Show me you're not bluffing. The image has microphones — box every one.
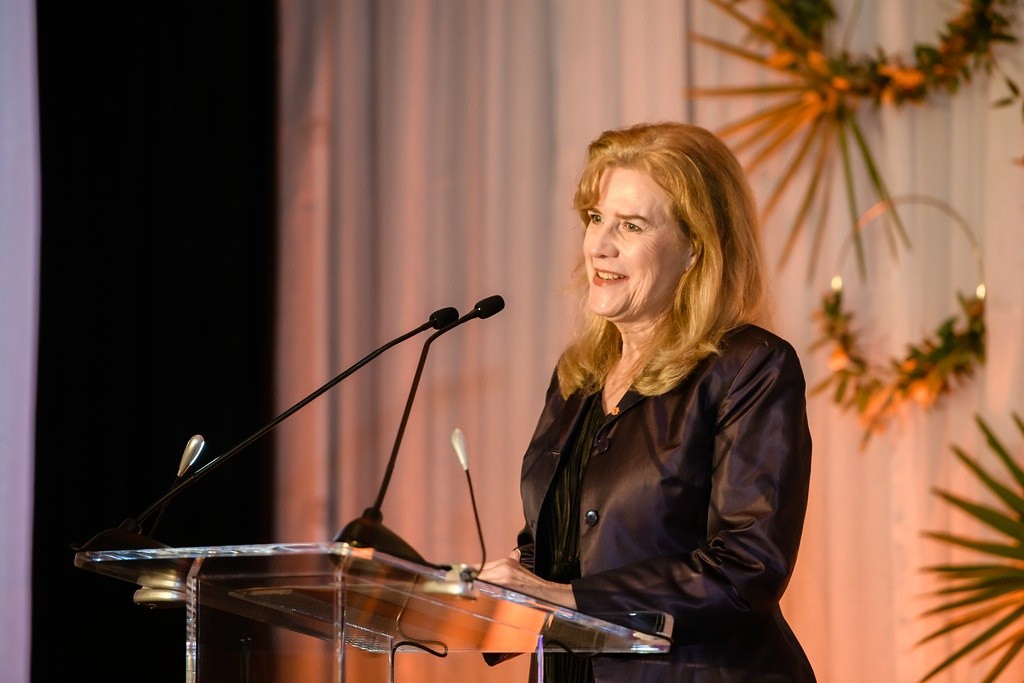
[78,305,460,567]
[329,294,505,580]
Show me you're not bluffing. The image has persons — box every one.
[458,120,822,683]
